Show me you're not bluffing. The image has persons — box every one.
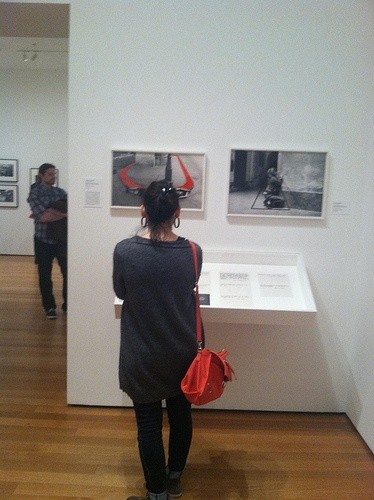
[27,162,68,320]
[112,180,206,500]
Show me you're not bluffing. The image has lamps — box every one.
[23,52,38,62]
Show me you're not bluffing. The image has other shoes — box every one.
[46,309,57,319]
[145,467,182,497]
[127,489,169,500]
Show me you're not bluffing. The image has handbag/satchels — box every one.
[179,347,238,406]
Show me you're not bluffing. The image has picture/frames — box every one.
[109,148,207,212]
[225,147,330,219]
[29,168,59,188]
[0,185,18,208]
[0,159,18,182]
